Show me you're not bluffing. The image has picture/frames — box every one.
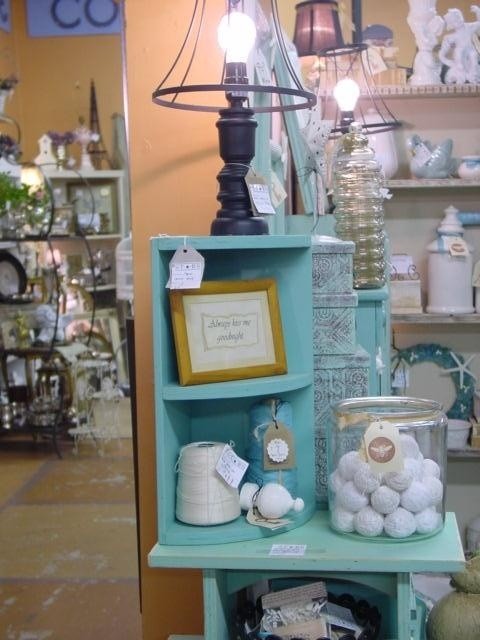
[165,278,286,386]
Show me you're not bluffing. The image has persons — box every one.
[436,4,480,84]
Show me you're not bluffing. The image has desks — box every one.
[148,510,468,639]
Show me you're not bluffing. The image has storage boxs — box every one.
[313,235,373,510]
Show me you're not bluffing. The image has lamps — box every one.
[151,0,318,235]
[318,43,401,136]
[293,1,345,57]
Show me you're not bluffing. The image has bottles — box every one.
[331,121,387,290]
[327,395,448,544]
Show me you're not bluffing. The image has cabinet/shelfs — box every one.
[359,290,393,398]
[284,79,478,461]
